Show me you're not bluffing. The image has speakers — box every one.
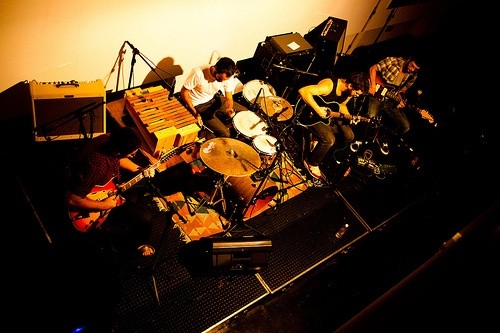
[318,17,348,68]
[200,237,273,276]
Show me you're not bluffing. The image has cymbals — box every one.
[198,136,261,178]
[260,97,294,120]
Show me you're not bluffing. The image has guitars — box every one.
[296,91,369,127]
[357,63,434,122]
[69,140,193,234]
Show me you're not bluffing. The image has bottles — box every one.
[335,223,349,238]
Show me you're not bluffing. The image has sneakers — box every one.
[303,159,322,180]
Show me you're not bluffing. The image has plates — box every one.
[138,245,157,256]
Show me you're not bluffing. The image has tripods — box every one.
[223,125,285,237]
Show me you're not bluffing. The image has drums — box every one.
[242,77,276,104]
[254,134,281,168]
[230,109,272,137]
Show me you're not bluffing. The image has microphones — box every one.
[168,79,177,100]
[254,89,262,104]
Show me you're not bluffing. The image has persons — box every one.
[181,57,249,139]
[351,55,422,156]
[298,71,369,180]
[65,128,156,257]
